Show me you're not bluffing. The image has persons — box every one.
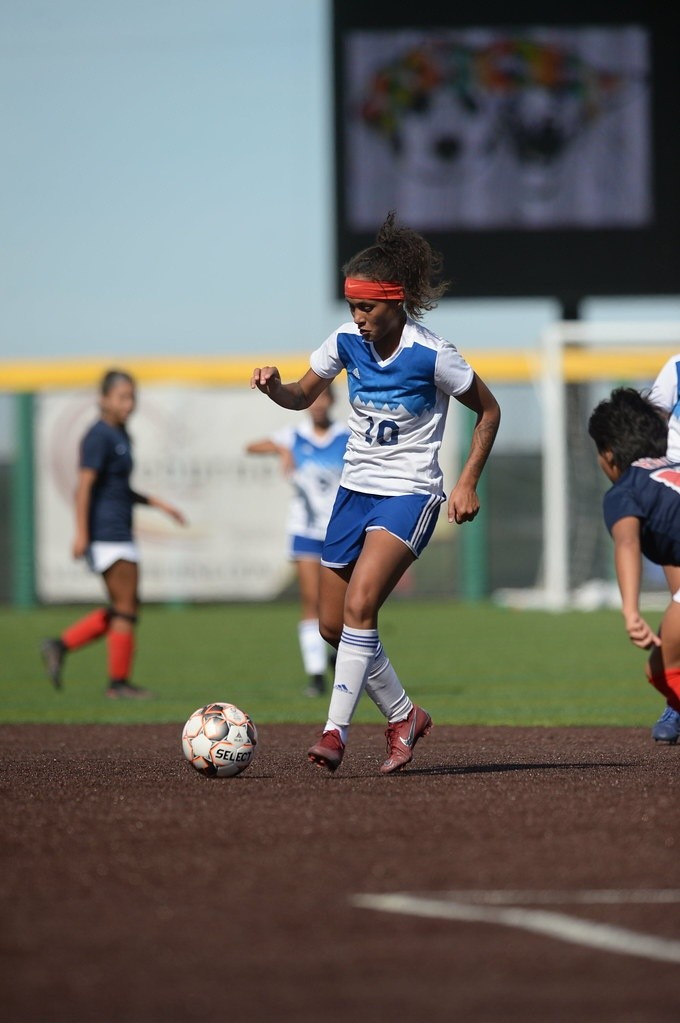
[250,208,502,777]
[643,354,680,744]
[245,385,353,697]
[39,371,187,699]
[588,386,680,719]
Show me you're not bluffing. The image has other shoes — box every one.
[109,683,153,700]
[41,642,66,688]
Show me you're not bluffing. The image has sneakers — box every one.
[308,730,345,774]
[651,705,680,746]
[381,705,434,774]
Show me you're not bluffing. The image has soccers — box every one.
[181,701,260,778]
